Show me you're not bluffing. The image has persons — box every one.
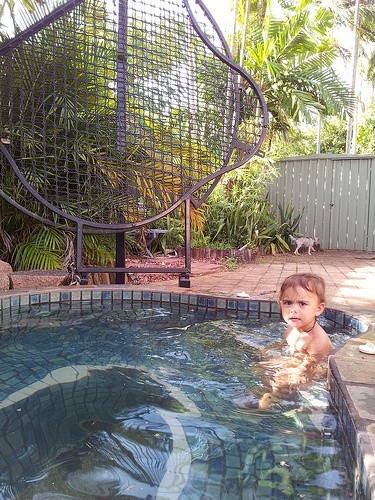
[236,273,335,409]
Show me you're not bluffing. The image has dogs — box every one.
[288,235,320,256]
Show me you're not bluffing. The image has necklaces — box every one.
[300,321,316,333]
